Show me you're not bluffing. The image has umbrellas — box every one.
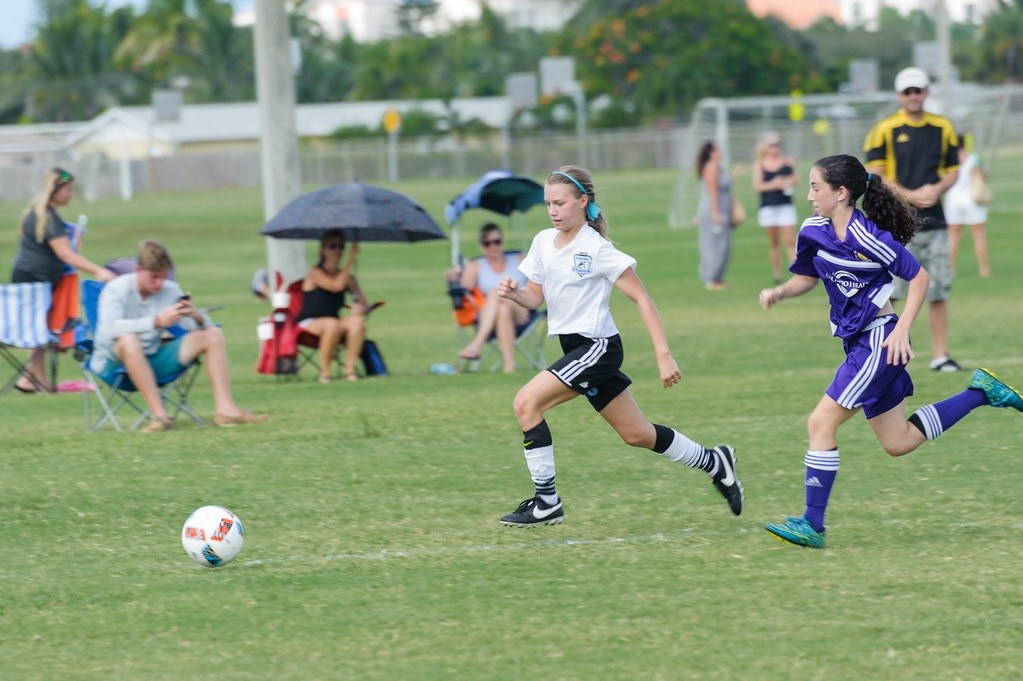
[259,178,446,243]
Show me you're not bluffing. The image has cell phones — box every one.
[179,296,189,300]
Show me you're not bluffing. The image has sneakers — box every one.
[499,495,564,529]
[967,368,1023,412]
[711,445,746,516]
[764,515,826,549]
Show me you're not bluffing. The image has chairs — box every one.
[448,250,548,373]
[0,256,222,433]
[269,279,386,382]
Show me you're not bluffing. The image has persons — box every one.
[752,132,798,284]
[695,139,745,289]
[446,223,532,373]
[864,66,961,374]
[90,240,268,434]
[760,154,1023,549]
[497,164,745,527]
[941,137,992,278]
[252,268,268,303]
[11,167,116,392]
[296,230,369,382]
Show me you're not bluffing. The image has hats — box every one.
[894,67,928,92]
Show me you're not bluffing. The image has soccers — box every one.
[181,505,244,567]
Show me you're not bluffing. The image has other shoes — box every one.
[930,360,961,371]
[457,351,479,359]
[140,417,174,434]
[214,409,270,428]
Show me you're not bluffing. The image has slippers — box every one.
[14,384,36,393]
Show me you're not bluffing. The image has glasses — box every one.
[323,243,346,250]
[482,239,501,247]
